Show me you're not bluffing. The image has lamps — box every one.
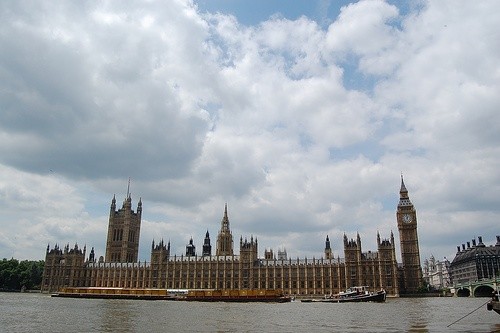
[402,213,413,223]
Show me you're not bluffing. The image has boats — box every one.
[51,292,60,297]
[186,289,292,301]
[301,299,312,302]
[322,286,387,303]
[61,287,170,298]
